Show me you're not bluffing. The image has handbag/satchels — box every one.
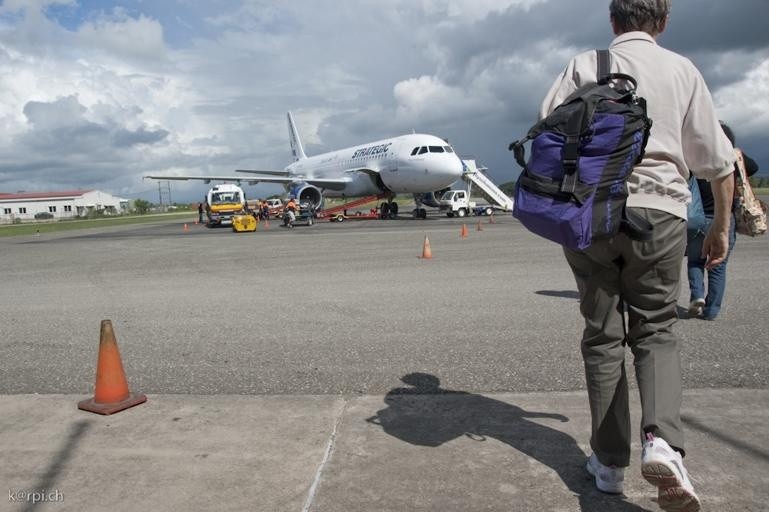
[687,175,707,239]
[732,147,769,237]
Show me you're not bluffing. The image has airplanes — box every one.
[138,108,488,221]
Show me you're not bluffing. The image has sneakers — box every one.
[640,433,702,511]
[586,450,626,494]
[689,298,704,317]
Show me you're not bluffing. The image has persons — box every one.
[198,203,205,223]
[259,198,297,229]
[686,121,758,321]
[539,0,737,511]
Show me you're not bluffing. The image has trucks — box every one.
[203,183,249,228]
[264,198,283,218]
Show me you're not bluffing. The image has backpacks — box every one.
[508,72,653,250]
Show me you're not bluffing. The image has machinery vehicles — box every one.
[438,157,514,218]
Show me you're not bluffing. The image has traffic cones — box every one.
[419,234,435,260]
[458,222,471,239]
[73,315,150,419]
[487,214,495,224]
[476,218,482,231]
[182,223,189,231]
[194,219,198,224]
